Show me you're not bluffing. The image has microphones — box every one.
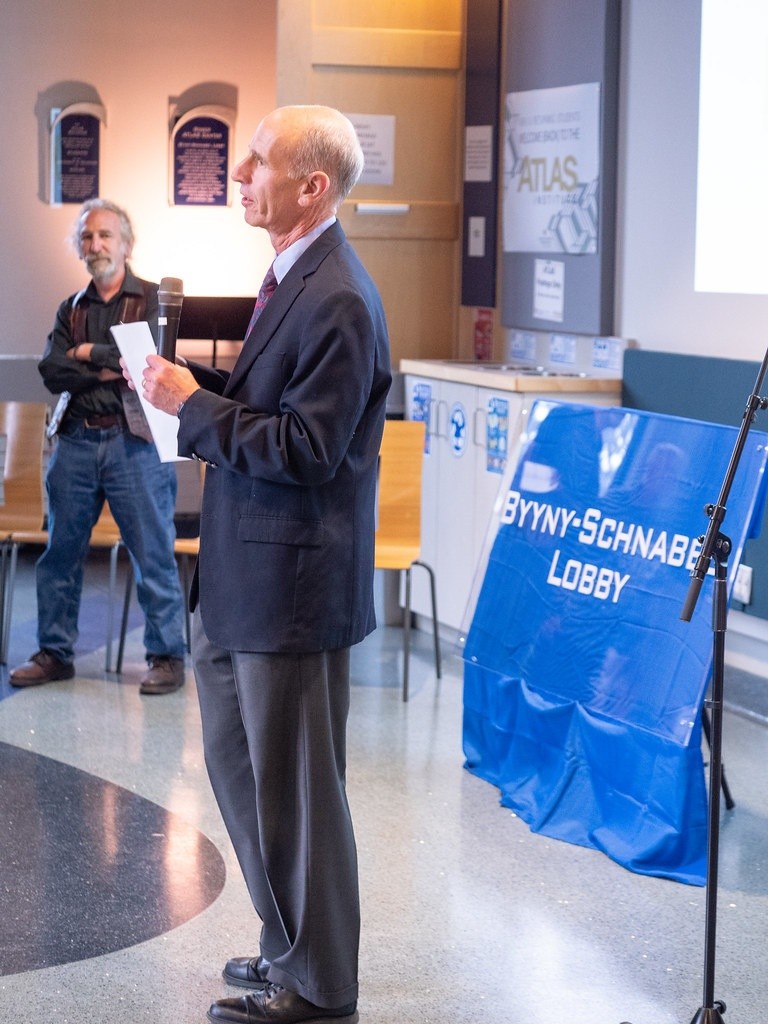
[157,277,185,367]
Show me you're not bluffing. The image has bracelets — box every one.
[174,399,187,420]
[72,341,86,360]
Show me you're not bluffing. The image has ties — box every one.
[243,263,278,343]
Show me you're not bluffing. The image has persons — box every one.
[118,106,391,1024]
[7,197,187,696]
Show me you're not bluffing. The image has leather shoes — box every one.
[8,650,74,686]
[206,981,359,1023]
[139,656,185,694]
[222,954,271,988]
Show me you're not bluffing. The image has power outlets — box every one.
[732,564,752,604]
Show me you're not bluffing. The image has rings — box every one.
[141,380,148,387]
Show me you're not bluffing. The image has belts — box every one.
[83,413,127,429]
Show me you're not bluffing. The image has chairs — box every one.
[0,401,440,703]
